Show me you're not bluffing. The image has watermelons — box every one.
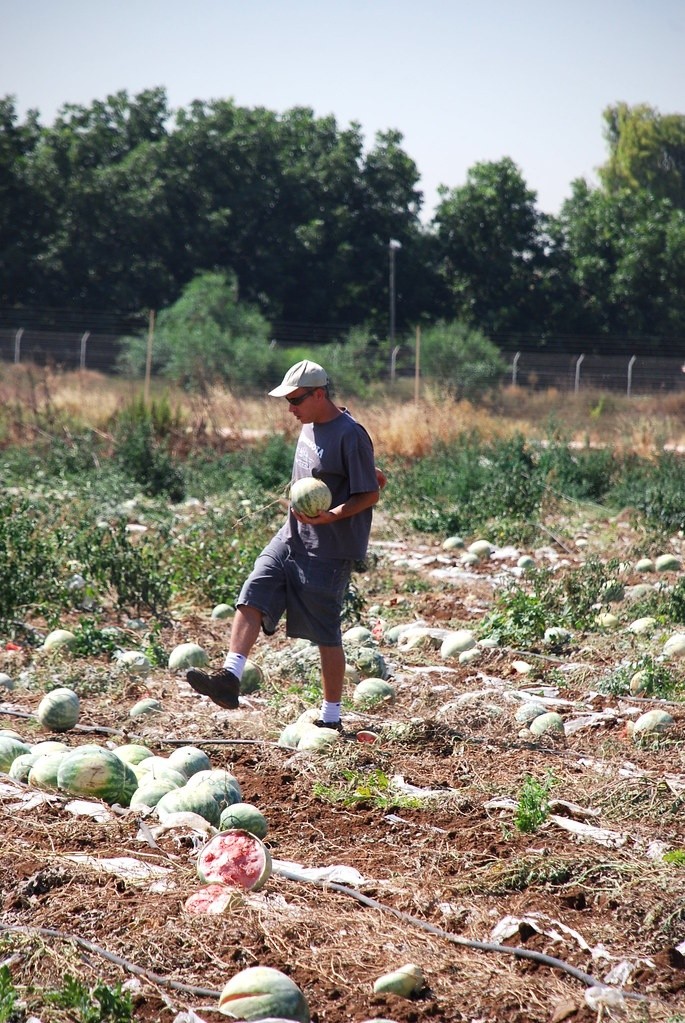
[0,477,685,1023]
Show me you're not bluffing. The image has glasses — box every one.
[285,387,318,406]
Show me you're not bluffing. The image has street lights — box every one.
[387,238,403,353]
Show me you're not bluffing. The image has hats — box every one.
[267,359,328,398]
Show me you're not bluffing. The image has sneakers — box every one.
[186,668,239,709]
[295,717,344,750]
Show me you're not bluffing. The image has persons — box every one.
[186,359,383,732]
